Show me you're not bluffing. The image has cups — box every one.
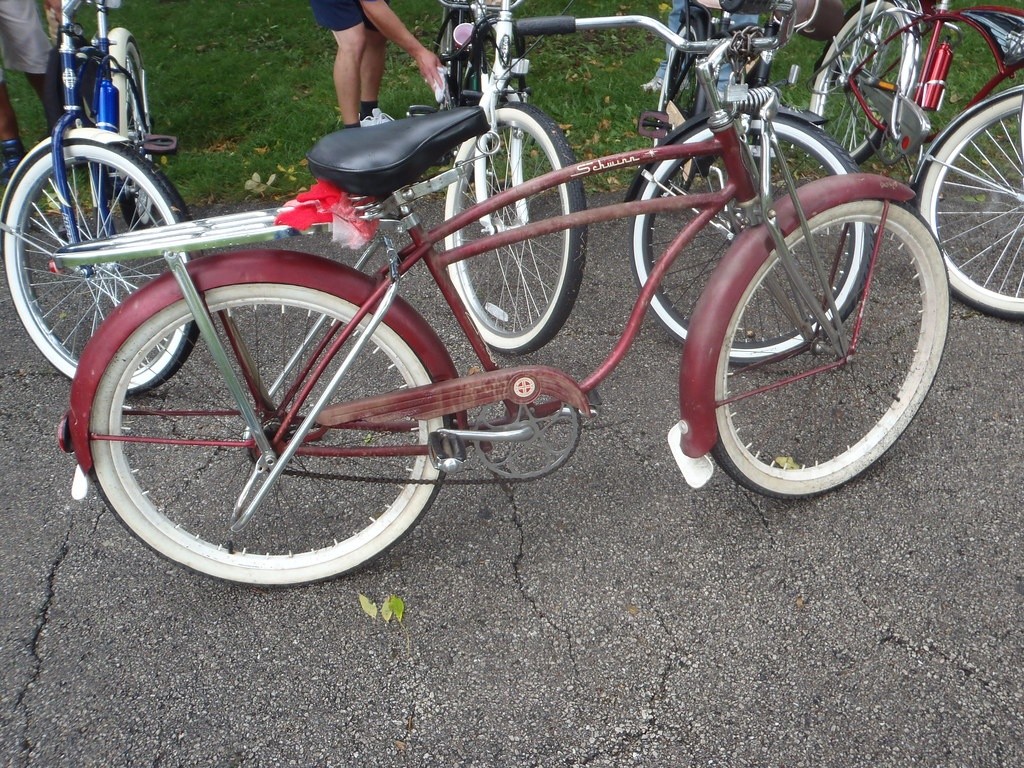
[453,23,474,48]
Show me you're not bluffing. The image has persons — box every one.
[0,0,61,186]
[309,0,444,129]
[641,0,759,102]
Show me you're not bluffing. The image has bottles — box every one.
[918,34,953,111]
[94,77,120,133]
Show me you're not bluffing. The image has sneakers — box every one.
[641,77,663,90]
[2,139,24,183]
[359,108,395,127]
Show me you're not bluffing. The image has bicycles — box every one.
[806,1,1024,322]
[404,1,588,356]
[621,0,873,368]
[46,15,951,592]
[0,0,207,398]
